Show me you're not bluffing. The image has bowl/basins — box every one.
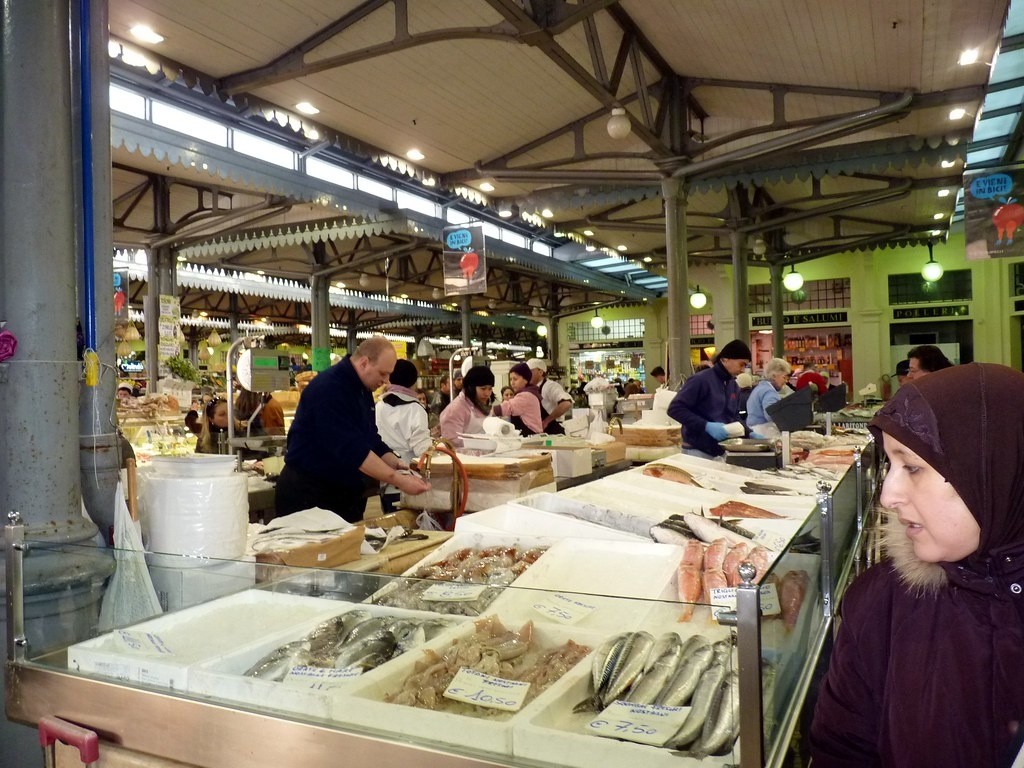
[719,438,775,450]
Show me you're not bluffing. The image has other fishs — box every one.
[641,430,871,633]
[570,630,777,760]
[243,609,458,685]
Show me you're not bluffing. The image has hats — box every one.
[792,368,802,375]
[389,359,418,388]
[453,369,462,380]
[736,373,752,388]
[890,359,909,377]
[820,371,828,377]
[720,340,752,362]
[462,366,494,387]
[119,382,133,394]
[527,358,547,373]
[509,363,532,381]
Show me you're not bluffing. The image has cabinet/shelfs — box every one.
[754,336,834,377]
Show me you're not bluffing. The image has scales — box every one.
[723,386,814,471]
[226,337,291,451]
[448,348,491,402]
[814,383,846,436]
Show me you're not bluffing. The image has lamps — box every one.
[784,263,804,291]
[607,107,632,139]
[690,284,706,308]
[921,47,992,281]
[105,23,603,338]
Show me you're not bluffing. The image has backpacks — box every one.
[234,394,276,461]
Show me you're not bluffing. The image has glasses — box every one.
[207,396,222,412]
[905,368,921,373]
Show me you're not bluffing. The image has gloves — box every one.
[748,432,763,439]
[705,421,728,441]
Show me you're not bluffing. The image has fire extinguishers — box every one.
[882,374,891,401]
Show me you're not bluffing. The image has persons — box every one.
[274,336,431,525]
[115,356,846,514]
[668,340,769,456]
[802,362,1024,768]
[891,346,954,387]
[746,358,791,437]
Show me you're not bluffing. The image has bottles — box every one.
[783,333,851,352]
[784,354,840,377]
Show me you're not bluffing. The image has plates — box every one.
[150,455,238,476]
